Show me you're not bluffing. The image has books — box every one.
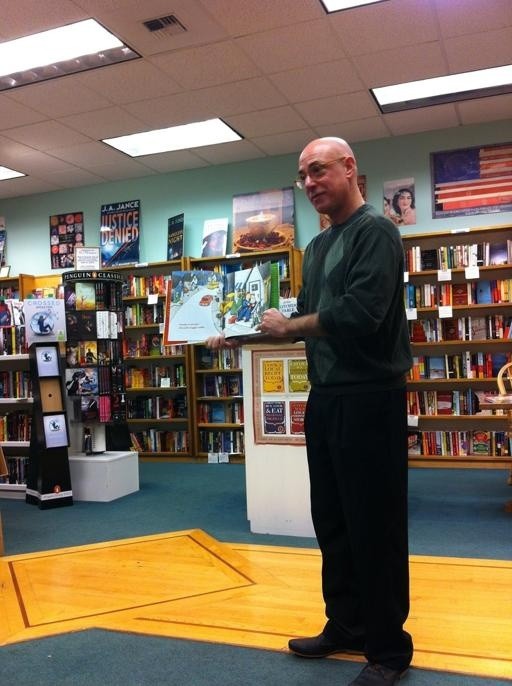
[0,250,298,488]
[400,235,512,458]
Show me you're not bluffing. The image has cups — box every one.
[246,214,276,235]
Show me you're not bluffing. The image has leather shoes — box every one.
[288,634,366,657]
[346,664,409,686]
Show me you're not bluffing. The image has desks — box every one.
[478,395,512,513]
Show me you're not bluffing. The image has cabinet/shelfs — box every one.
[397,226,511,469]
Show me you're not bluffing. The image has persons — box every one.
[254,137,416,685]
[384,187,415,226]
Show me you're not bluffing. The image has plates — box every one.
[234,231,288,251]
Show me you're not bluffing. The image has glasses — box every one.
[293,157,341,190]
